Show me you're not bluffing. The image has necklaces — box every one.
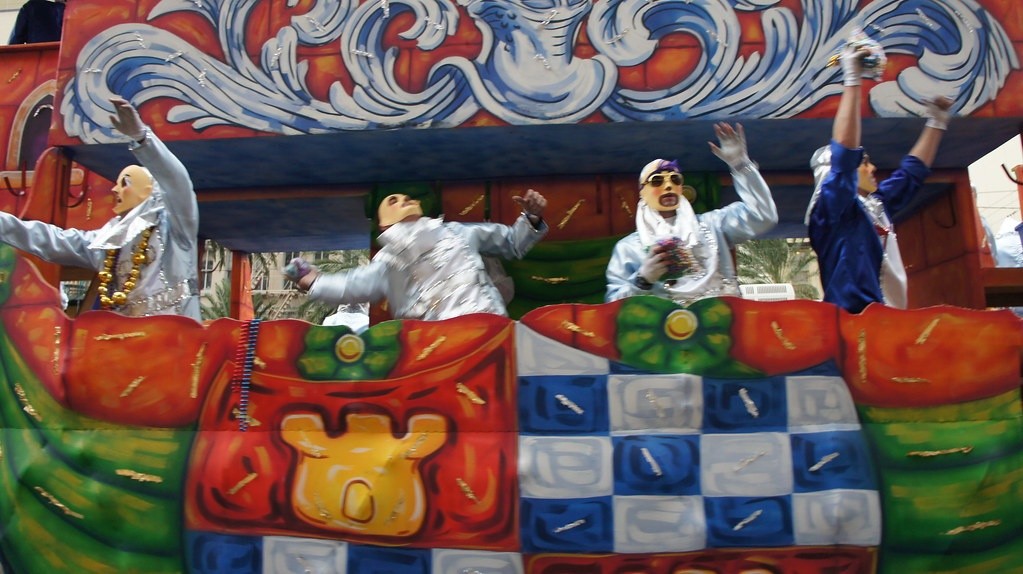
[658,237,693,278]
[98,230,150,309]
[827,38,887,76]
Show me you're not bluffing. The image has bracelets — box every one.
[521,208,541,218]
[732,164,756,178]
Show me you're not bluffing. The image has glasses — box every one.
[640,173,683,185]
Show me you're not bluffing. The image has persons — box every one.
[804,38,954,314]
[604,121,779,308]
[0,99,204,325]
[281,184,550,322]
